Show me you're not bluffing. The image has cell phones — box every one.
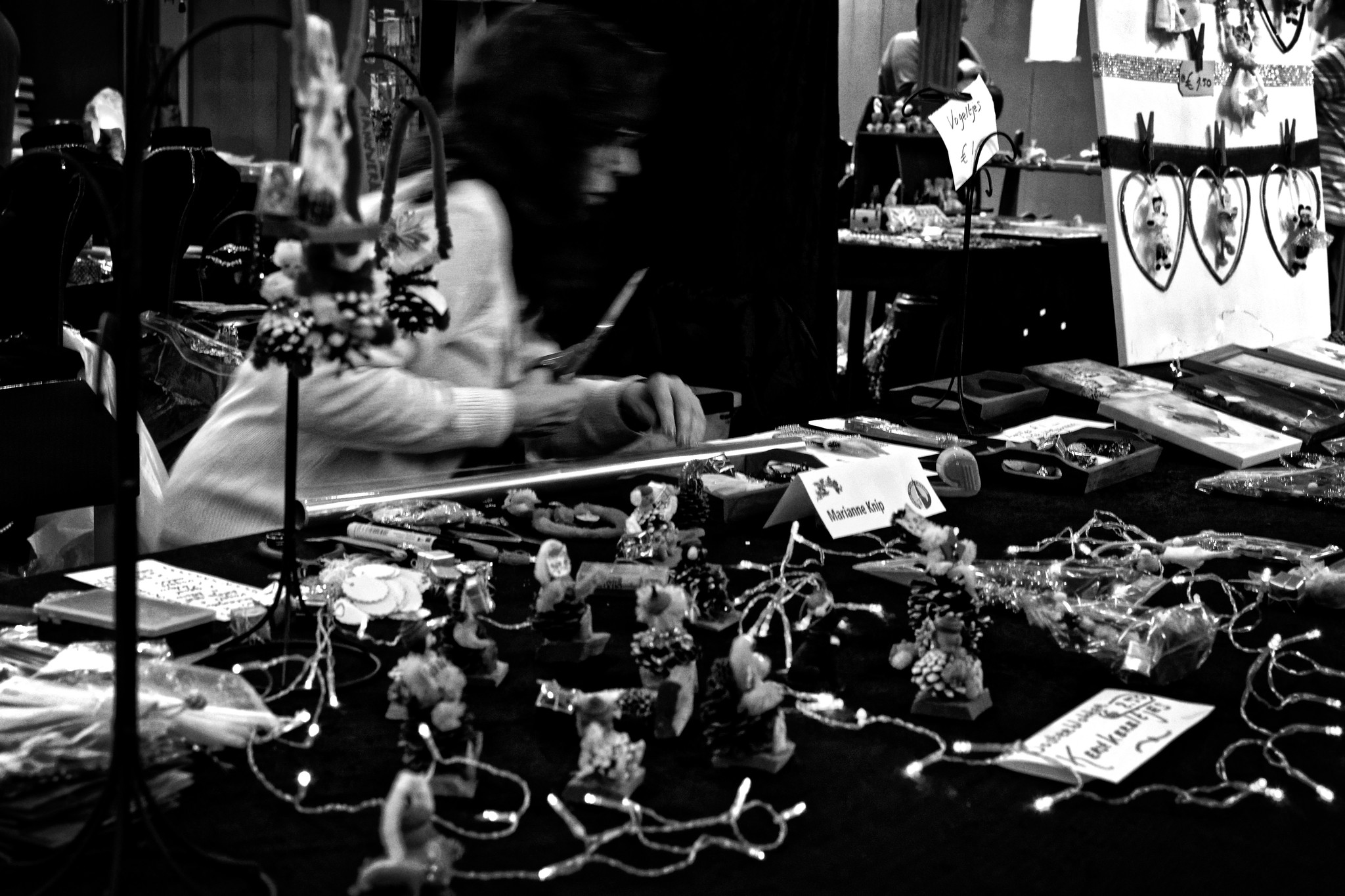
[33,587,218,638]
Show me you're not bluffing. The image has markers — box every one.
[347,521,442,553]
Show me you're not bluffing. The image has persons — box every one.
[161,0,705,549]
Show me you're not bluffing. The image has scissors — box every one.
[522,267,646,378]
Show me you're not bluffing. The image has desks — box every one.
[0,341,1345,896]
[836,233,1120,417]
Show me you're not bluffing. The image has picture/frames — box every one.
[1184,342,1345,406]
